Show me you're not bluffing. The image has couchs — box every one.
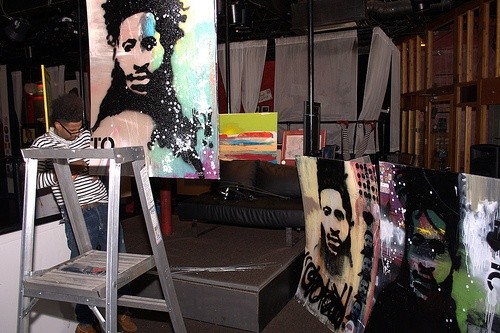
[178,196,303,227]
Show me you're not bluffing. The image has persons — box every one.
[30,92,138,333]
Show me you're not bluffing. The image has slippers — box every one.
[117,312,139,333]
[75,323,96,333]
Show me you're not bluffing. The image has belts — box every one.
[81,202,100,210]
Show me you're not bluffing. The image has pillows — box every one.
[218,159,260,198]
[256,160,302,199]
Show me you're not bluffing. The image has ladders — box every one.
[16,146,187,333]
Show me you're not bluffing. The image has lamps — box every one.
[366,0,429,19]
[0,0,32,43]
[217,0,252,35]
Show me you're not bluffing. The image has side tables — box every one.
[177,179,212,196]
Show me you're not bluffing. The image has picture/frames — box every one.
[261,106,270,112]
[256,105,260,112]
[281,129,326,166]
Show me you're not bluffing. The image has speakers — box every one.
[303,101,321,156]
[470,144,500,179]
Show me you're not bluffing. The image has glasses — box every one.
[55,119,86,138]
